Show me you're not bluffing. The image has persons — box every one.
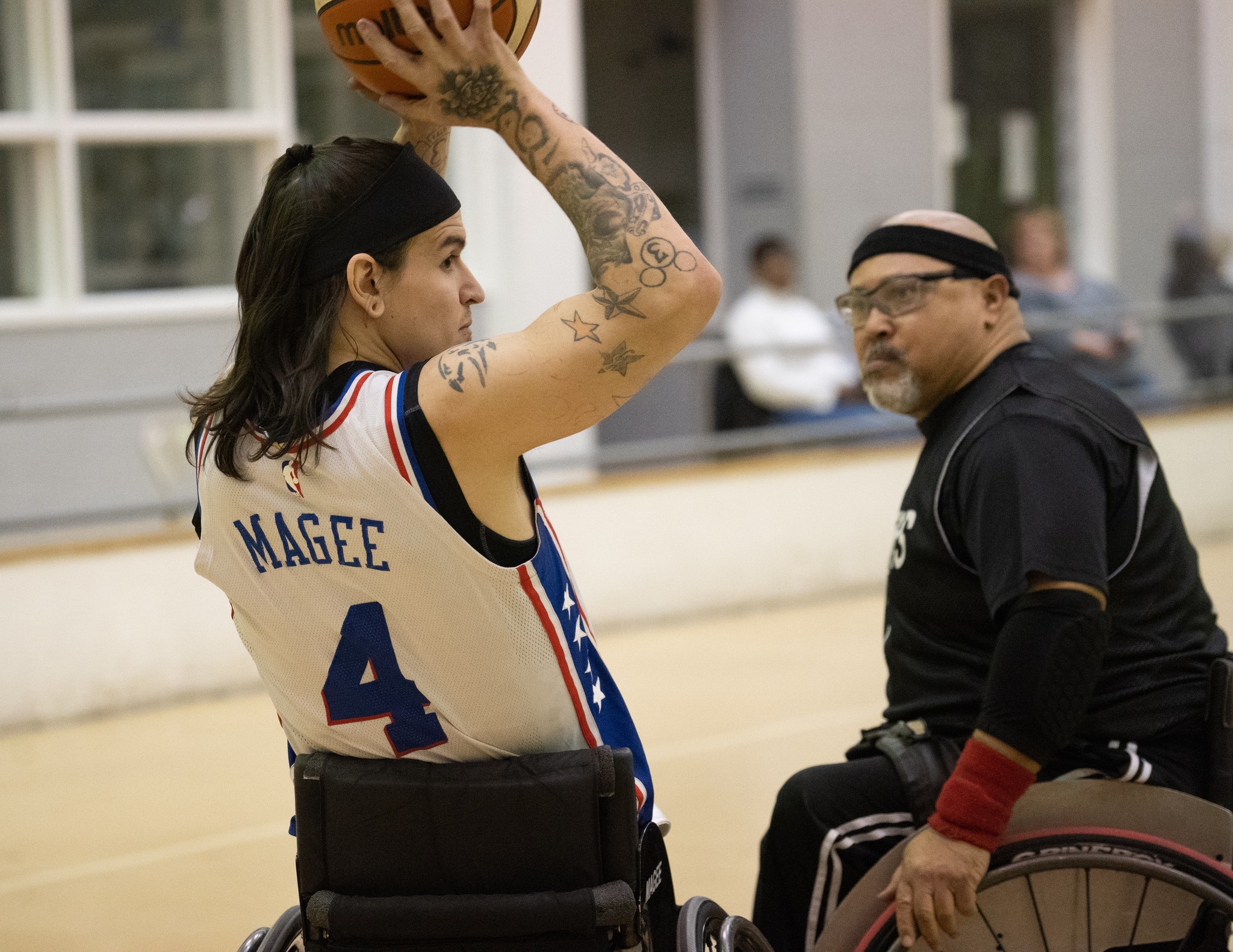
[1162,221,1233,381]
[180,0,725,951]
[723,230,872,425]
[1004,204,1153,391]
[750,209,1233,951]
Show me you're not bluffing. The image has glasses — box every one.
[835,267,967,328]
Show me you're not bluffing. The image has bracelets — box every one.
[927,738,1036,852]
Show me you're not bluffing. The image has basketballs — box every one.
[313,0,543,99]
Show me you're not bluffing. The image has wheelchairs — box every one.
[810,647,1233,952]
[237,743,774,952]
